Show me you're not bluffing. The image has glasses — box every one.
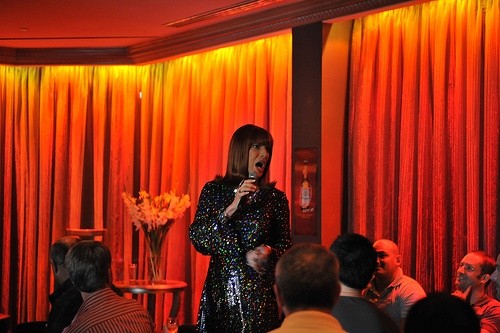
[459,263,484,276]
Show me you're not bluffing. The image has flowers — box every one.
[121,182,192,282]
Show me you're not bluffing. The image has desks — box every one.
[112,278,188,333]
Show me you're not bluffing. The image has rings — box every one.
[239,179,245,187]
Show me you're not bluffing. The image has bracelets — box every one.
[265,244,271,250]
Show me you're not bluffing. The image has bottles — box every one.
[298,160,310,212]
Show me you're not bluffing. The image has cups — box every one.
[128,264,137,285]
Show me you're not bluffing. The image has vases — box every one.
[147,255,163,284]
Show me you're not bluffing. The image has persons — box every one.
[62,240,156,333]
[46,235,84,333]
[265,242,349,333]
[329,231,500,333]
[188,123,294,333]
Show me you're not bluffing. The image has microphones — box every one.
[246,173,257,197]
